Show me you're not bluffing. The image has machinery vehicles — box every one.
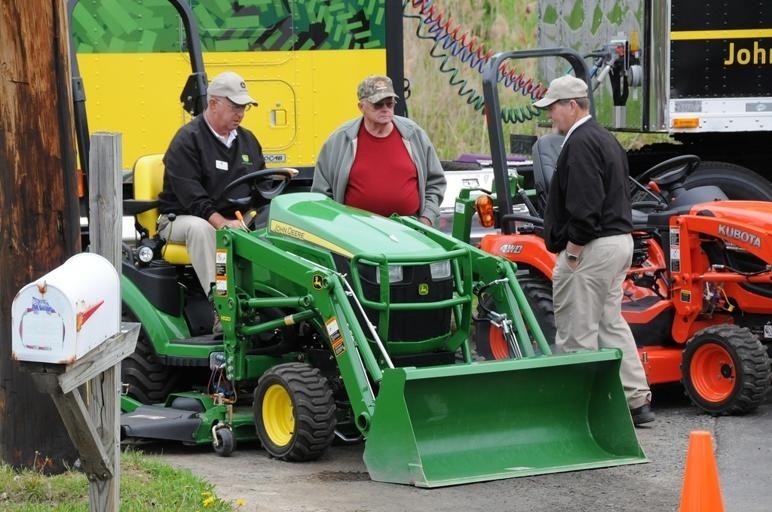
[121,0,652,492]
[467,38,772,416]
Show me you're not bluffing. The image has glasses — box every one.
[218,100,253,113]
[373,101,396,110]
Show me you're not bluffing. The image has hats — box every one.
[357,75,399,103]
[532,76,588,108]
[207,72,253,106]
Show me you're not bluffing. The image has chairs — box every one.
[531,133,652,225]
[132,153,196,266]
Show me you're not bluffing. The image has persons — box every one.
[532,74,656,427]
[310,74,448,227]
[158,72,269,339]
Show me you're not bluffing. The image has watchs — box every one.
[564,251,580,262]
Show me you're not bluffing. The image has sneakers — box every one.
[631,405,655,424]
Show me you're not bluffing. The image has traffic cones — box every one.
[679,432,728,512]
